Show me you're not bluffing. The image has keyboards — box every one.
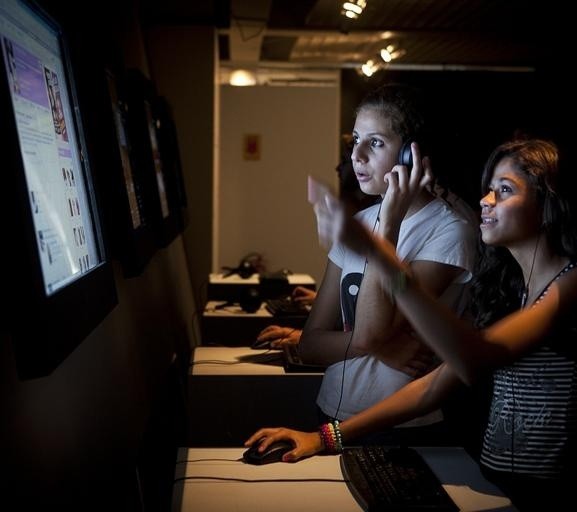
[267,299,308,317]
[282,344,327,373]
[340,446,461,512]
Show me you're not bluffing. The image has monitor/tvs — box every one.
[0,0,118,383]
[156,96,188,213]
[67,26,159,279]
[123,67,186,249]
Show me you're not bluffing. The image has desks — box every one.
[203,298,311,346]
[209,272,316,299]
[192,344,326,449]
[182,448,510,512]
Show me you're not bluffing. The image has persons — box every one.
[297,83,478,443]
[292,285,318,305]
[256,324,303,347]
[244,139,577,512]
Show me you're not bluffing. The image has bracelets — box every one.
[319,420,344,455]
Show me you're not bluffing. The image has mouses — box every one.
[251,337,280,350]
[243,439,294,465]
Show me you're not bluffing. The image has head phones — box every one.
[398,142,413,166]
[221,254,263,279]
[215,286,262,313]
